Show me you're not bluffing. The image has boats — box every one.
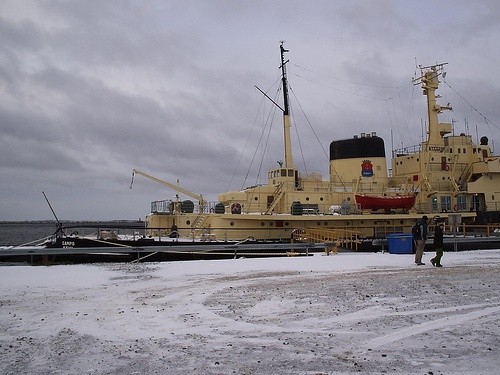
[354,195,417,211]
[43,40,500,242]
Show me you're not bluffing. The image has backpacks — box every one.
[412,225,421,240]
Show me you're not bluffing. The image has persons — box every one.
[430,223,445,267]
[411,215,429,265]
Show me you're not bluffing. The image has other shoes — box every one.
[418,263,425,265]
[436,264,442,267]
[430,259,435,266]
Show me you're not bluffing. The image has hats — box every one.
[421,216,428,220]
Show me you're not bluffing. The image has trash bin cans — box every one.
[385,233,414,254]
